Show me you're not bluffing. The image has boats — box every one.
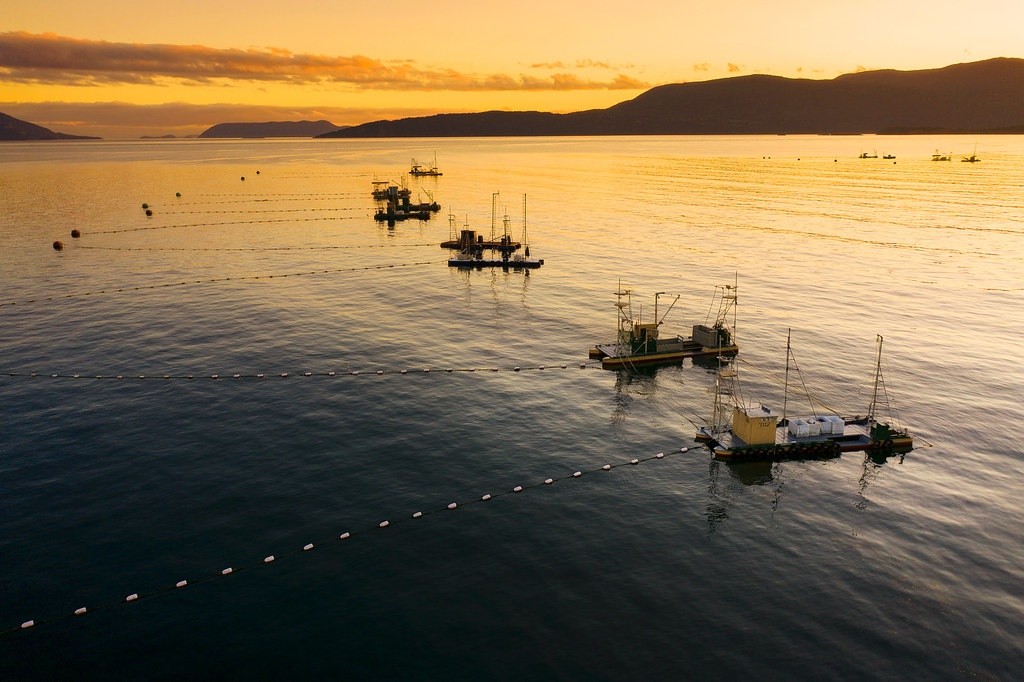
[409,150,443,176]
[449,190,545,268]
[589,269,739,372]
[371,181,442,222]
[960,155,981,162]
[440,192,522,253]
[859,151,879,158]
[883,154,895,159]
[931,149,951,161]
[696,326,914,463]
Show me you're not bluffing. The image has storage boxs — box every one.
[828,417,845,434]
[811,417,832,435]
[802,418,820,436]
[788,419,809,437]
